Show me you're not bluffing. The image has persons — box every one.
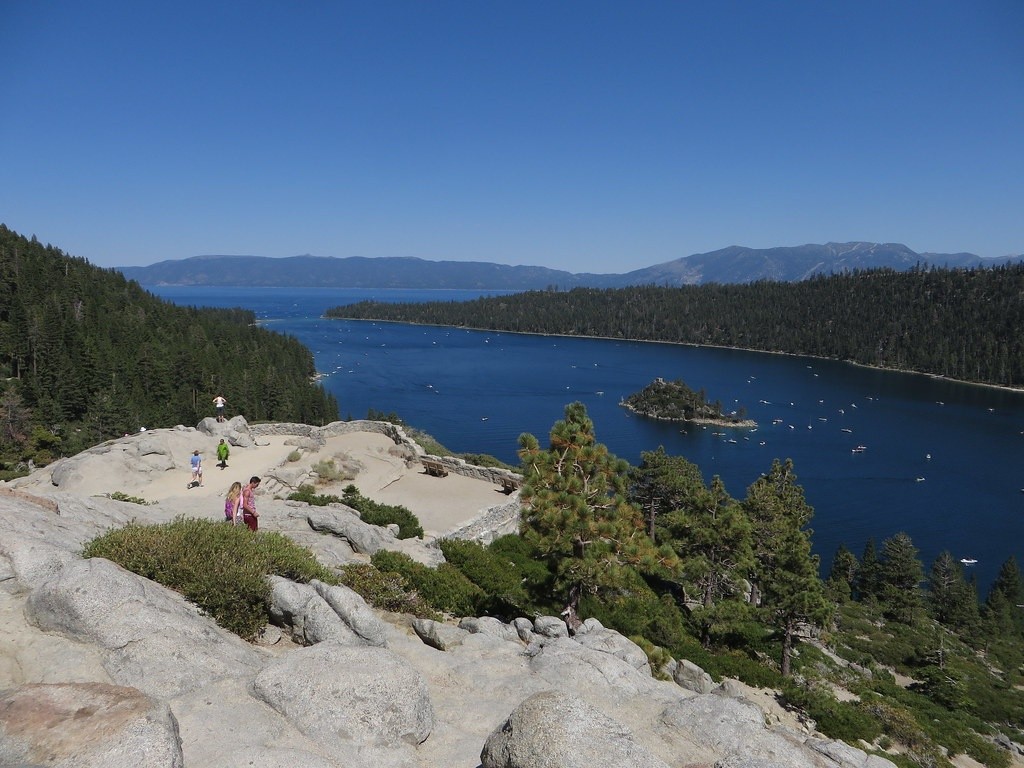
[217,439,230,470]
[212,394,227,423]
[224,482,242,526]
[242,476,261,532]
[188,450,206,487]
[139,426,147,432]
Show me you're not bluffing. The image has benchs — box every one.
[421,459,450,477]
[500,477,521,495]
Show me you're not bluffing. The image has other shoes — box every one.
[199,484,204,487]
[221,467,224,470]
[187,484,190,489]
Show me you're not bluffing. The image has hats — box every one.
[192,450,201,454]
[140,427,146,431]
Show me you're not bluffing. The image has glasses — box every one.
[256,483,258,486]
